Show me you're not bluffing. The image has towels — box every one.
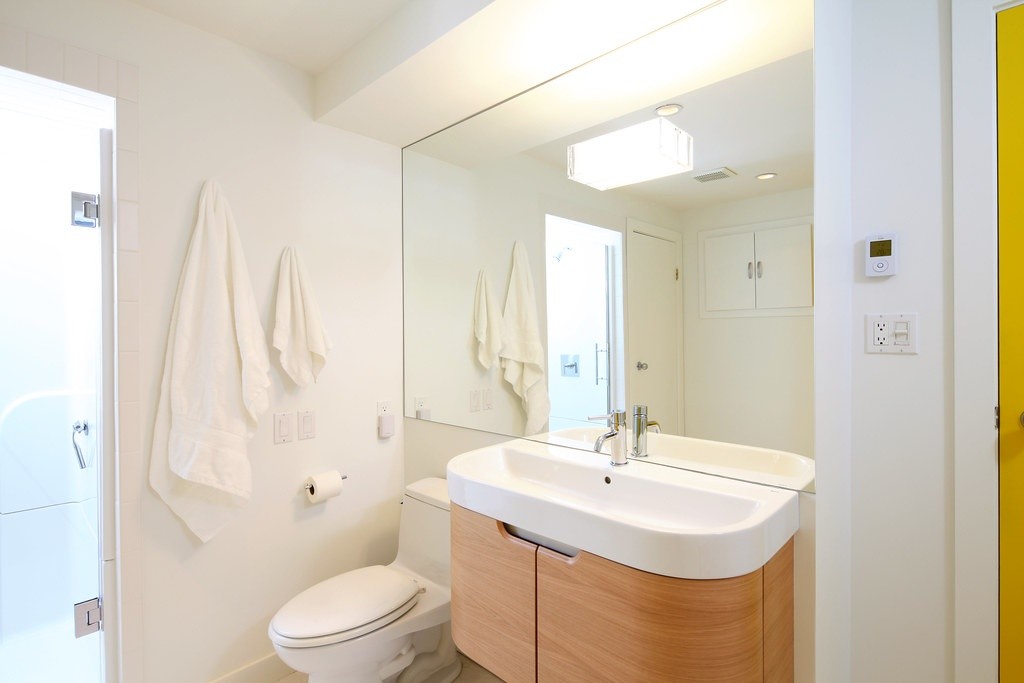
[147,173,272,544]
[499,238,549,437]
[474,267,509,371]
[272,247,325,389]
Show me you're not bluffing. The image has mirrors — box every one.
[399,0,814,494]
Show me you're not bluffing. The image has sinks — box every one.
[543,424,815,492]
[445,438,801,581]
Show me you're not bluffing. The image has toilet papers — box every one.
[303,470,343,505]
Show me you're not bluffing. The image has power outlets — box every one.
[865,312,919,355]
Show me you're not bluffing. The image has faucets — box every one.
[630,405,661,458]
[594,409,629,466]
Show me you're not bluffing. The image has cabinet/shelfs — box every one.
[695,216,815,318]
[448,502,795,683]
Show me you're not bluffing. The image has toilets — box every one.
[267,476,463,682]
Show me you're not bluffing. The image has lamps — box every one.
[567,117,697,192]
